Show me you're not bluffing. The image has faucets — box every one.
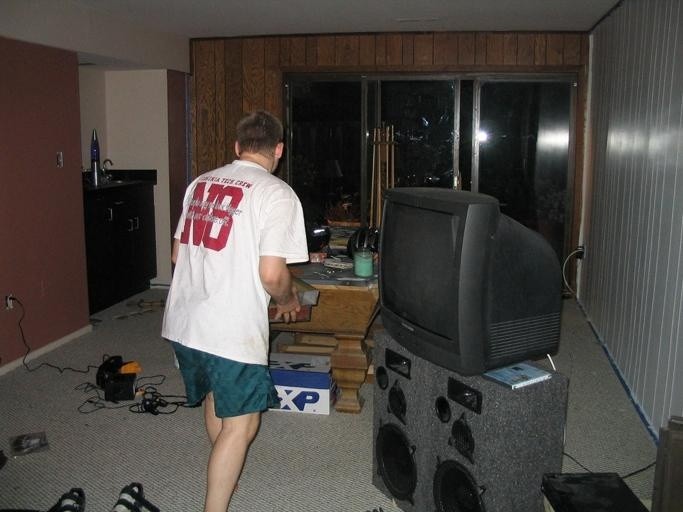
[103,159,112,177]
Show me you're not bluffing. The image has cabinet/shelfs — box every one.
[84,187,120,316]
[114,182,159,316]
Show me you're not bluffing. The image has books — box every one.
[482,360,553,391]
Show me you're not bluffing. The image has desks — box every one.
[371,329,570,508]
[266,221,381,414]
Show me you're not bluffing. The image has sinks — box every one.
[112,179,139,184]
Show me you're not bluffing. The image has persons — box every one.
[49,480,144,511]
[155,109,310,512]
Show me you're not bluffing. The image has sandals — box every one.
[110,480,163,512]
[44,485,86,512]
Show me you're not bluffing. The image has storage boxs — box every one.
[264,330,337,415]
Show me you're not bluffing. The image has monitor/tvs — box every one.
[376,186,564,380]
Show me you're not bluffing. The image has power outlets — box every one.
[6,294,14,311]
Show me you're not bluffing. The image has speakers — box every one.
[371,329,569,512]
